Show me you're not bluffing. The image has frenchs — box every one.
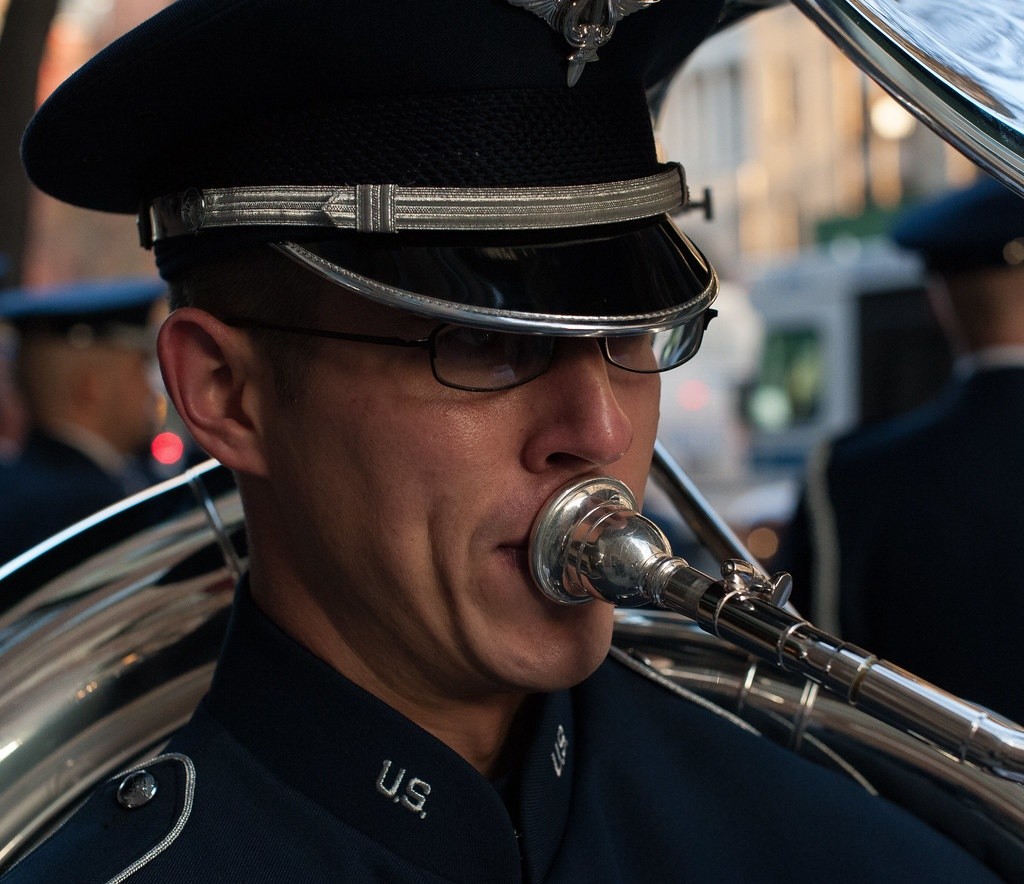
[0,2,1023,884]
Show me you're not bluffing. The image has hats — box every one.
[20,0,723,336]
[893,180,1024,269]
[0,278,171,334]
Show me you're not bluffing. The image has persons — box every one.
[0,279,249,615]
[0,1,1024,884]
[772,180,1023,787]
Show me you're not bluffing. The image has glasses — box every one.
[219,306,719,393]
